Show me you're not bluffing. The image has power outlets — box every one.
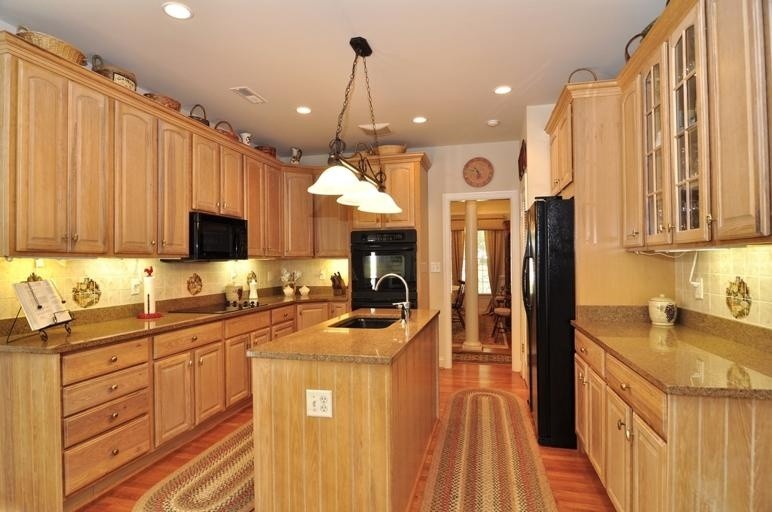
[694,278,703,299]
[306,390,333,417]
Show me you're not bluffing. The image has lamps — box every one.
[306,37,403,216]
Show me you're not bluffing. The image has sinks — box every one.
[327,314,400,329]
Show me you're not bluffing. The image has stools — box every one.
[491,296,511,343]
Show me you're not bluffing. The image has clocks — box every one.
[464,157,493,188]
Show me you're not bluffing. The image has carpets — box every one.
[421,388,556,512]
[133,417,255,512]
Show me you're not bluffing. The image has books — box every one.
[12,279,73,332]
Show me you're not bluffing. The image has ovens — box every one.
[351,242,416,291]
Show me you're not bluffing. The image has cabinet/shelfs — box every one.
[543,81,676,306]
[349,152,433,309]
[617,0,772,245]
[573,328,604,490]
[0,336,154,512]
[0,30,350,258]
[155,301,350,464]
[604,352,772,512]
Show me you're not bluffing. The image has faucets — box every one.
[374,273,409,301]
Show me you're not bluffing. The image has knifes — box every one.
[330,272,342,288]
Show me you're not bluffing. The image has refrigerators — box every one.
[520,197,574,447]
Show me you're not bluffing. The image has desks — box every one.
[452,285,460,304]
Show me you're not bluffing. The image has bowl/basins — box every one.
[375,145,406,154]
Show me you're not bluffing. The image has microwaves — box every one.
[189,210,250,263]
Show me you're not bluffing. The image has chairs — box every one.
[452,280,465,329]
